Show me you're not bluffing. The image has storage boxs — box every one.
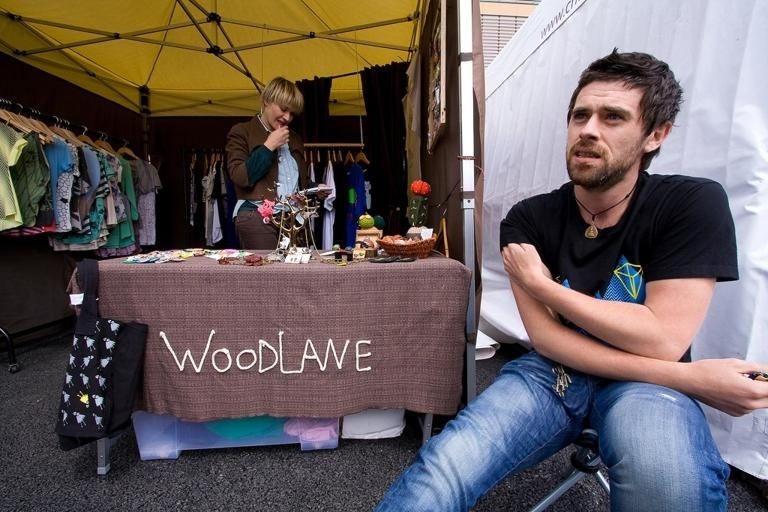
[130,413,339,461]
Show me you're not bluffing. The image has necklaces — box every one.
[575,182,635,238]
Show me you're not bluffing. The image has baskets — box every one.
[375,234,436,258]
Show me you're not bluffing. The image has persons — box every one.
[366,48,768,511]
[224,76,334,249]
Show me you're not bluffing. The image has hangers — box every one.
[189,147,224,168]
[302,141,370,166]
[0,97,142,162]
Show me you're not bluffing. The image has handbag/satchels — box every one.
[54,258,148,453]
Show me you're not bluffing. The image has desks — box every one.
[96,249,472,476]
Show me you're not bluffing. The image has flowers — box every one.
[405,179,431,226]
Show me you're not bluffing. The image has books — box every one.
[298,187,333,195]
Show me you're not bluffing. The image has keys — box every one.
[550,362,571,394]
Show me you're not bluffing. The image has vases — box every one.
[407,227,429,240]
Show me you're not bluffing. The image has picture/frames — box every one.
[425,1,446,155]
